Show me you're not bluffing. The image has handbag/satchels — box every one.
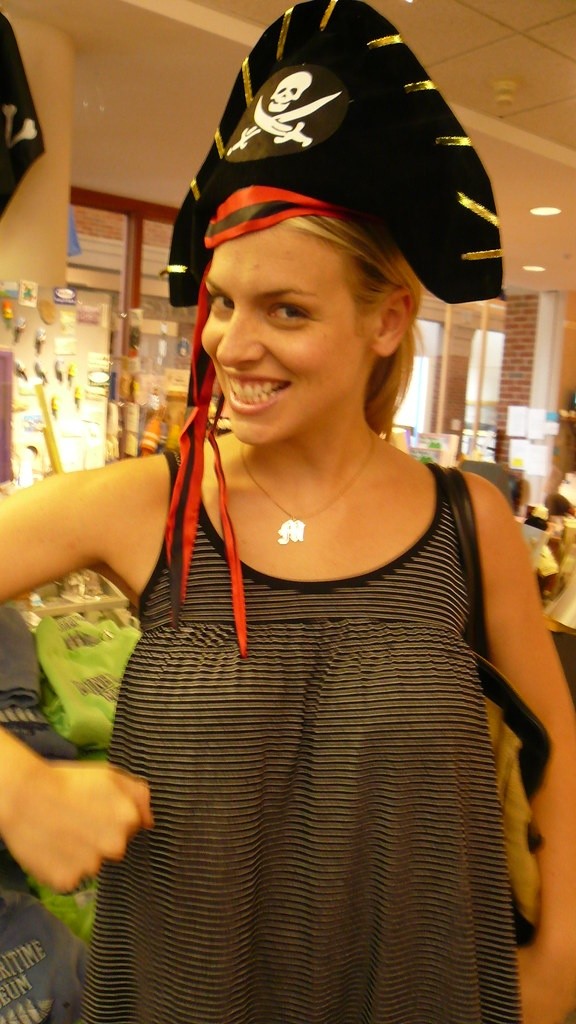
[435,459,555,945]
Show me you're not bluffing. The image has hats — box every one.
[168,0,506,307]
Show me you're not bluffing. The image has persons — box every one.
[0,184,576,1024]
[506,473,576,600]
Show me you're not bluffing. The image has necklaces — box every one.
[240,429,375,545]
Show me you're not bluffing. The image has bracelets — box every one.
[542,590,553,598]
